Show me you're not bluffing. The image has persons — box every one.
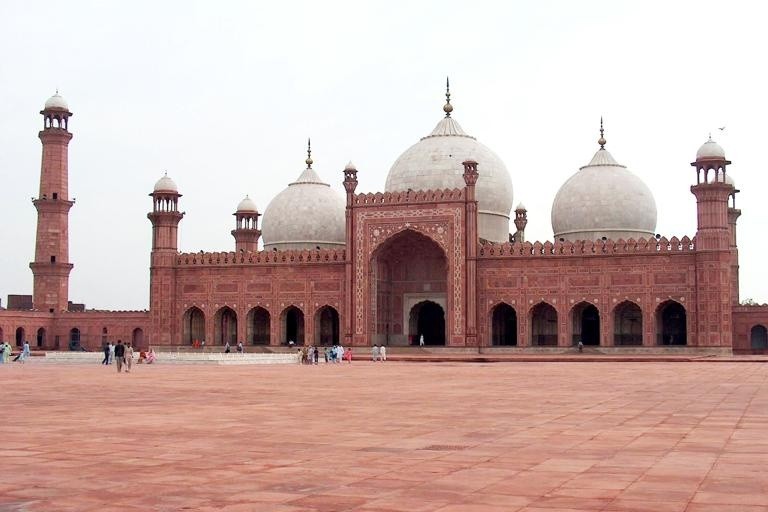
[237,341,243,354]
[192,338,206,350]
[371,344,386,362]
[224,342,231,353]
[578,340,583,352]
[0,341,30,364]
[298,344,352,364]
[420,334,424,347]
[102,339,155,373]
